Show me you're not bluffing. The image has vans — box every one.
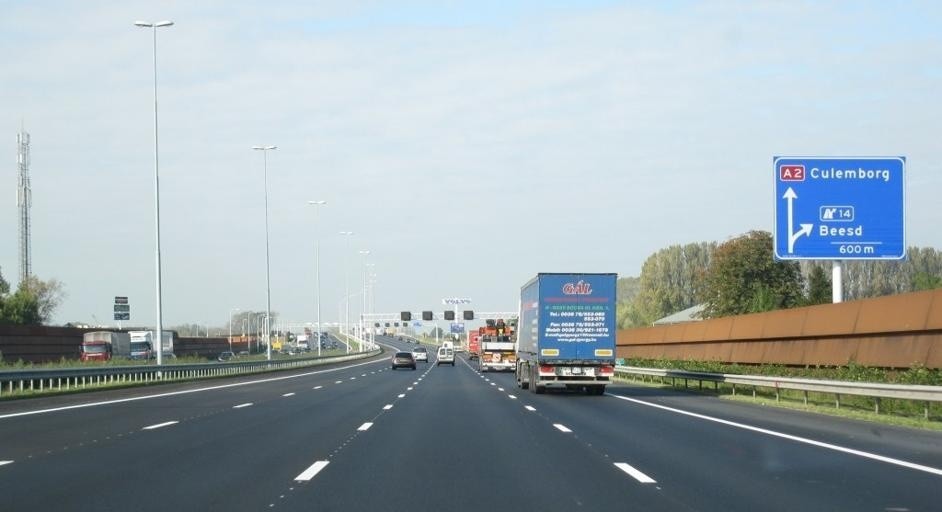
[436,346,456,366]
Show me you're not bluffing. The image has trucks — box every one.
[468,329,479,360]
[80,331,132,363]
[296,335,310,350]
[511,271,618,395]
[475,319,518,372]
[126,330,175,360]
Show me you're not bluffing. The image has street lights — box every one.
[135,18,179,377]
[228,307,275,353]
[252,145,278,367]
[338,229,353,355]
[360,249,378,352]
[307,200,326,357]
[442,297,472,341]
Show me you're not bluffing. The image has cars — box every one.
[217,351,249,362]
[264,344,311,357]
[314,332,338,351]
[384,331,421,344]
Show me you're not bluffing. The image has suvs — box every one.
[391,351,417,370]
[412,346,429,363]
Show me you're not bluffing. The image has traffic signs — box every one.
[773,156,905,263]
